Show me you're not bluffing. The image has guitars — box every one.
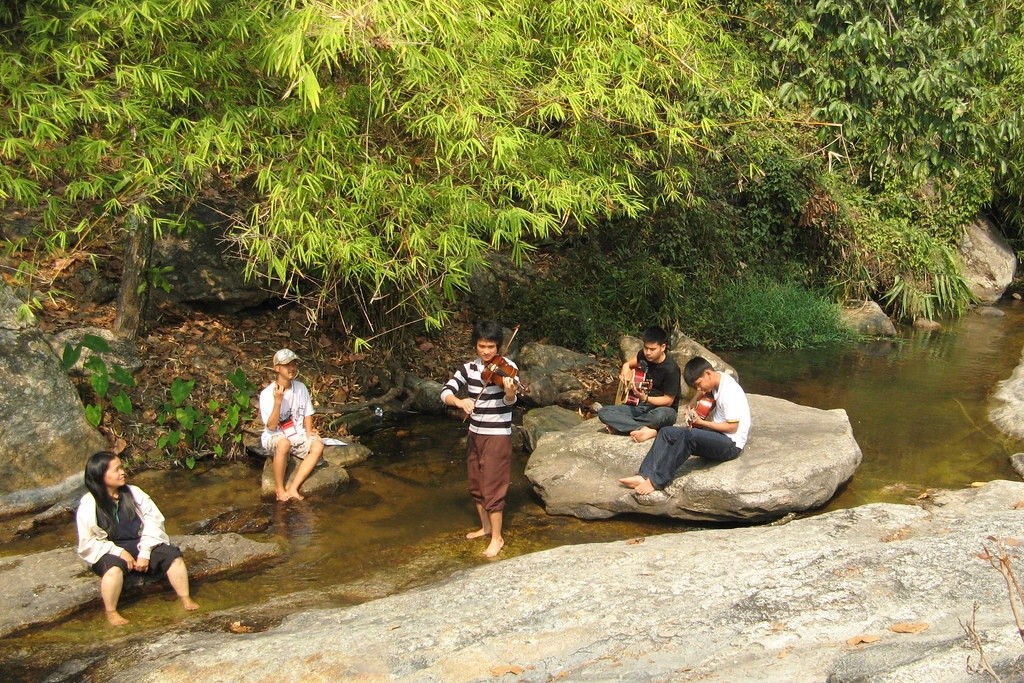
[685,393,716,426]
[614,370,653,404]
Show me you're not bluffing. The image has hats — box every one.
[273,348,300,365]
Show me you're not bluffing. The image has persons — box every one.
[259,348,324,501]
[598,325,682,443]
[76,452,196,624]
[619,357,751,495]
[440,320,520,557]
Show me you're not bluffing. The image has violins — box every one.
[480,354,531,393]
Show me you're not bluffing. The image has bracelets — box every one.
[643,395,648,404]
